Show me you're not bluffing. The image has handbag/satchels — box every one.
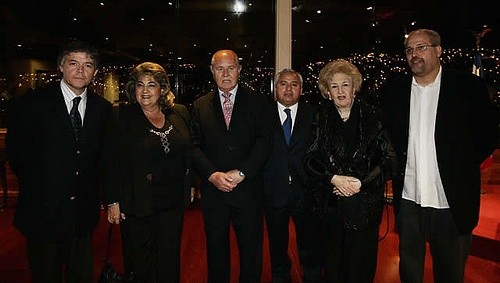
[99,261,137,283]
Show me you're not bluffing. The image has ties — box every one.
[282,109,292,146]
[69,97,82,144]
[221,93,234,131]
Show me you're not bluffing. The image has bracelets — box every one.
[108,202,116,207]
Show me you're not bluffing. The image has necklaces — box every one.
[149,125,174,154]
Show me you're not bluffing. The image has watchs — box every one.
[239,171,244,176]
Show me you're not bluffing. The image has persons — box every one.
[380,29,500,283]
[98,61,193,283]
[193,49,274,283]
[303,59,398,283]
[264,69,323,283]
[5,41,114,283]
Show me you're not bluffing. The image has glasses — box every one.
[404,44,437,55]
